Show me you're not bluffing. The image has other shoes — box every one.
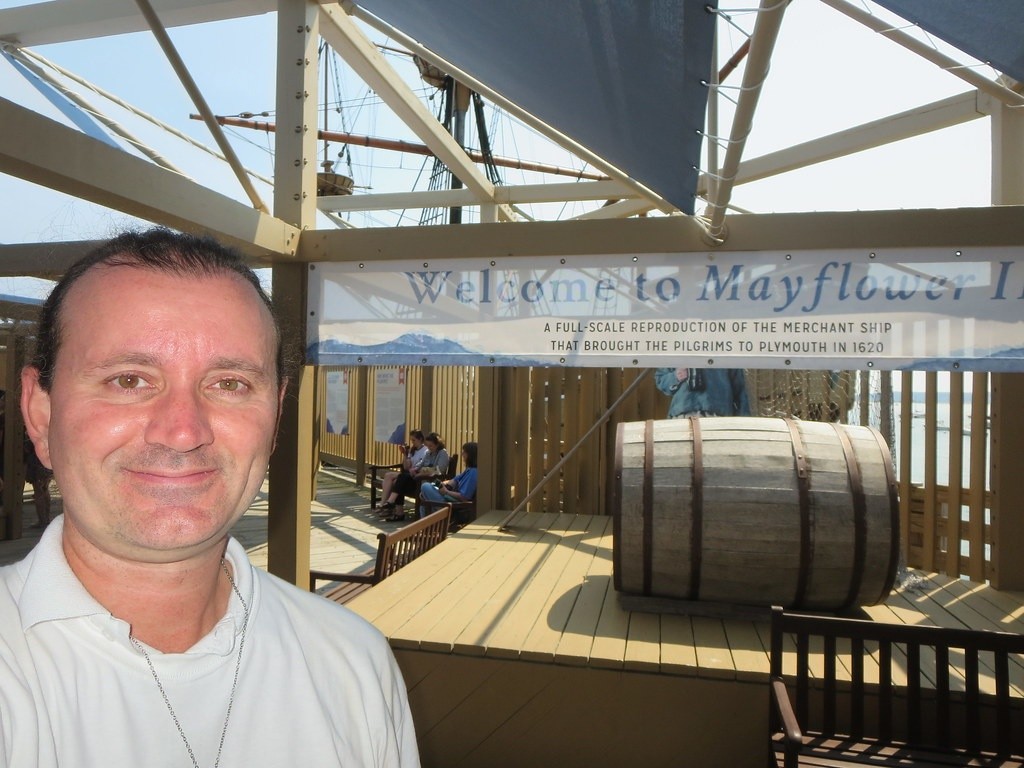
[385,514,405,522]
[379,510,393,518]
[379,502,396,509]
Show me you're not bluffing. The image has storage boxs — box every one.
[611,416,902,627]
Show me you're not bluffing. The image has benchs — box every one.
[309,502,452,605]
[367,443,459,519]
[764,604,1024,768]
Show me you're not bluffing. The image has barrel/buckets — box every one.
[609,415,902,610]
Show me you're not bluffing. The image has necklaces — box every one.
[125,556,250,768]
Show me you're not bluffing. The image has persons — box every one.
[0,226,427,768]
[655,367,752,420]
[419,442,479,537]
[377,429,449,522]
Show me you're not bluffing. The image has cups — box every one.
[683,367,704,391]
